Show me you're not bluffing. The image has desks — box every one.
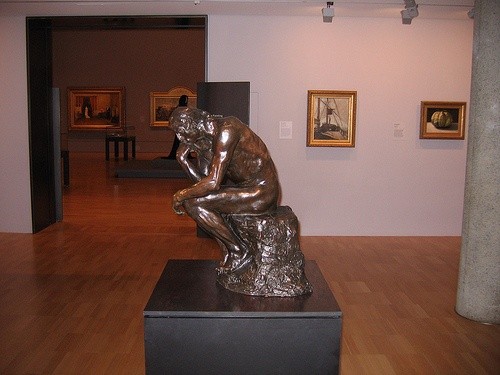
[106,136,135,161]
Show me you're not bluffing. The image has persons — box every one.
[168,94,193,159]
[169,106,279,272]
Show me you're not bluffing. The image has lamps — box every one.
[401,0,418,20]
[322,2,334,17]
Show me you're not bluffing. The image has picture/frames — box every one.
[420,100,467,140]
[67,87,127,134]
[149,90,197,127]
[307,90,355,147]
